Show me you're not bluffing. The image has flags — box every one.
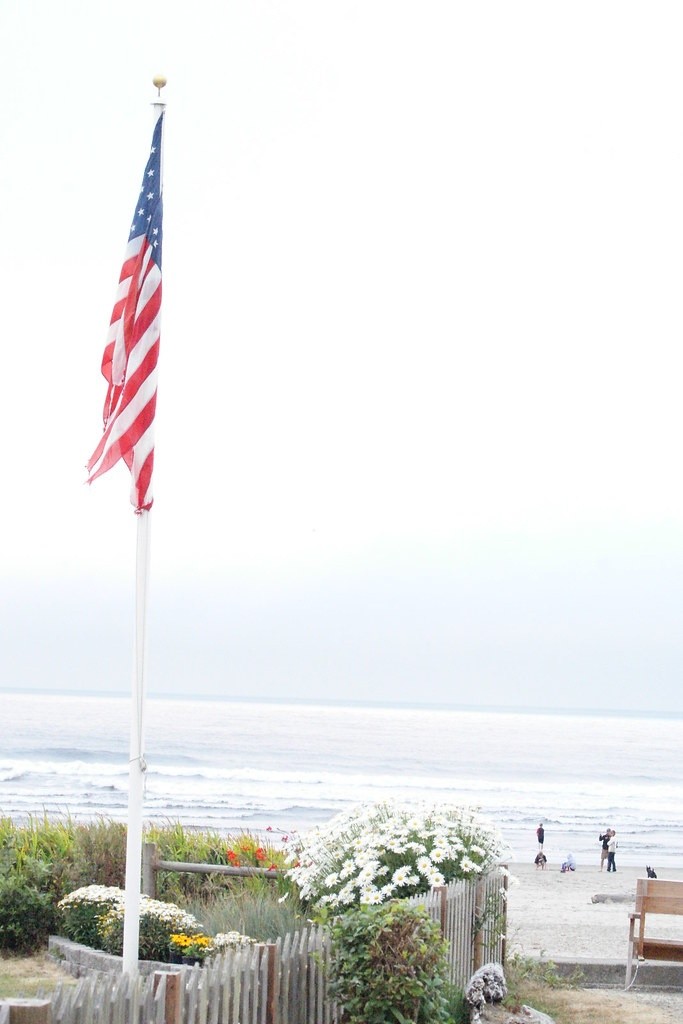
[84,97,168,516]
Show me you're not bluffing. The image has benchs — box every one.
[626,878,683,989]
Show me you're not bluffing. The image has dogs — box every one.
[646,864,657,878]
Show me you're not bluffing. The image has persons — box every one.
[534,852,547,871]
[560,854,577,873]
[537,823,545,849]
[597,828,617,872]
